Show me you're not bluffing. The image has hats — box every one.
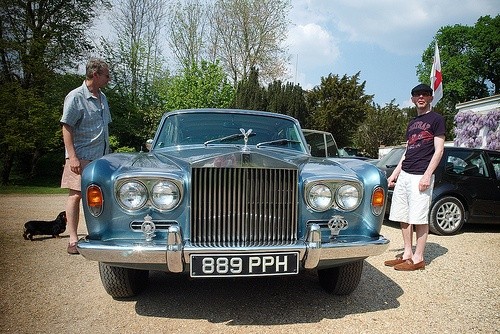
[411,84,432,95]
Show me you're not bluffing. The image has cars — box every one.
[76,109,390,298]
[377,148,500,235]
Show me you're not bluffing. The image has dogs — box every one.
[22,211,68,240]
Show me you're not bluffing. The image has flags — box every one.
[430,40,444,107]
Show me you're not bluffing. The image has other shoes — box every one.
[67,242,79,253]
[385,256,425,271]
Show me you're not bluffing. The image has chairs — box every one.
[446,161,483,177]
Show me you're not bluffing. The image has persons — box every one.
[59,57,112,254]
[384,83,446,271]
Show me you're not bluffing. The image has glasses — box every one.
[413,93,430,97]
[97,71,109,78]
